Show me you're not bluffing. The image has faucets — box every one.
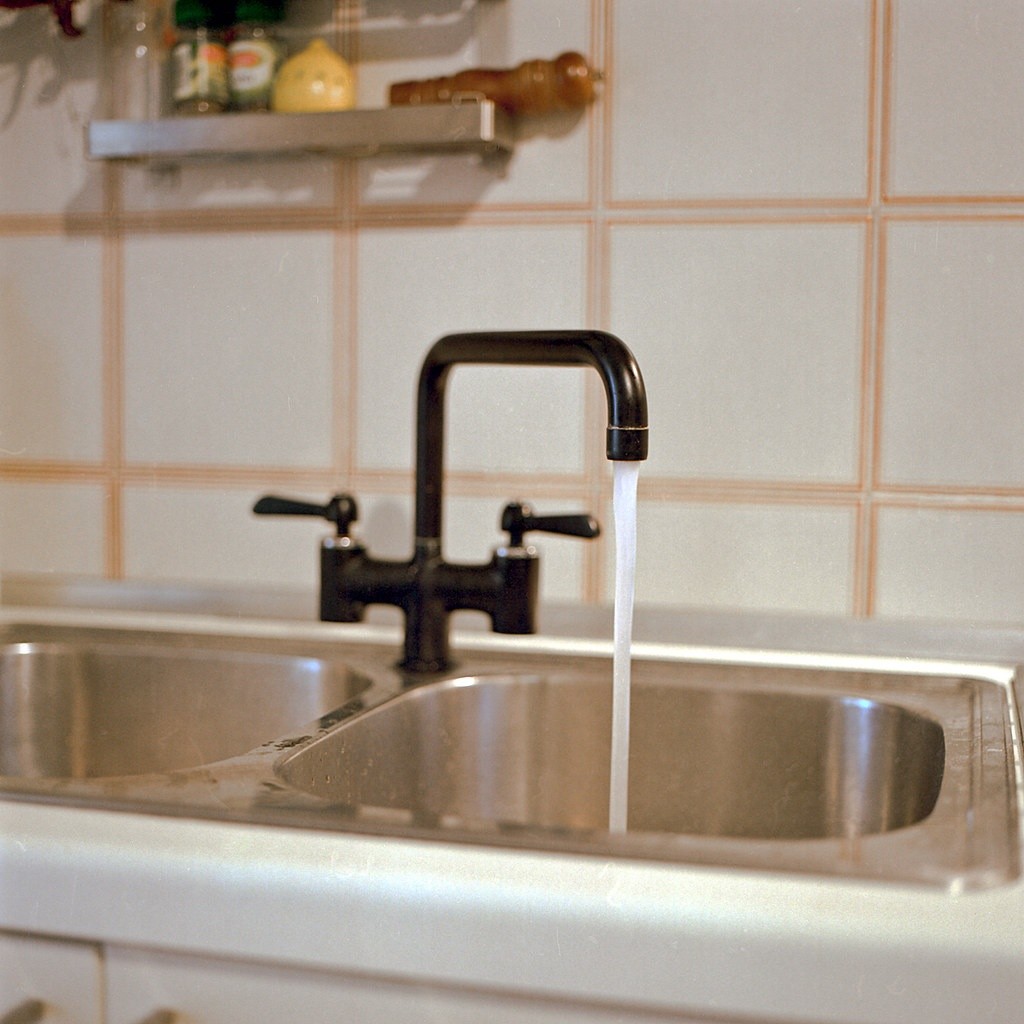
[242,327,651,671]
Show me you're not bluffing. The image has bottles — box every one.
[227,0,290,112]
[159,0,229,120]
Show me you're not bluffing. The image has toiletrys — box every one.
[106,3,606,114]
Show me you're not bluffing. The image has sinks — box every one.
[278,649,1024,901]
[0,620,377,828]
